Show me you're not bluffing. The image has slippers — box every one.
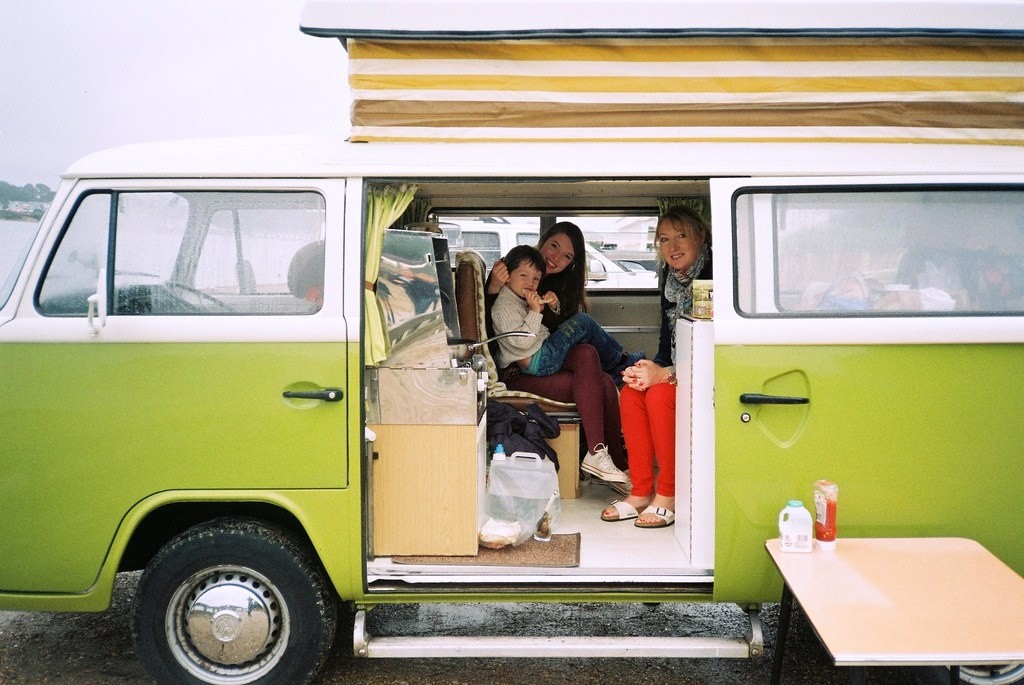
[633,504,675,528]
[601,499,652,521]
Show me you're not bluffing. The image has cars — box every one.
[436,218,659,288]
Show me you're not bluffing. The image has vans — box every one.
[0,1,1024,683]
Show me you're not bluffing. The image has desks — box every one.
[764,535,1024,685]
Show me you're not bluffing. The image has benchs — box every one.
[452,252,586,498]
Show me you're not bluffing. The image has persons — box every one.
[490,246,645,386]
[484,221,634,497]
[600,205,714,529]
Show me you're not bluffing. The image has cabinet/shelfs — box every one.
[365,409,488,556]
[675,312,715,568]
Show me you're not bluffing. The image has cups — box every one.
[691,278,714,318]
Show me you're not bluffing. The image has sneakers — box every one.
[581,443,629,483]
[605,469,633,497]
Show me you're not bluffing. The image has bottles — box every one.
[813,480,837,551]
[486,444,553,532]
[779,499,813,553]
[451,357,459,368]
[472,348,487,407]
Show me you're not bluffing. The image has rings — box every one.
[638,379,640,385]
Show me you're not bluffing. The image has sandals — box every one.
[612,350,647,386]
